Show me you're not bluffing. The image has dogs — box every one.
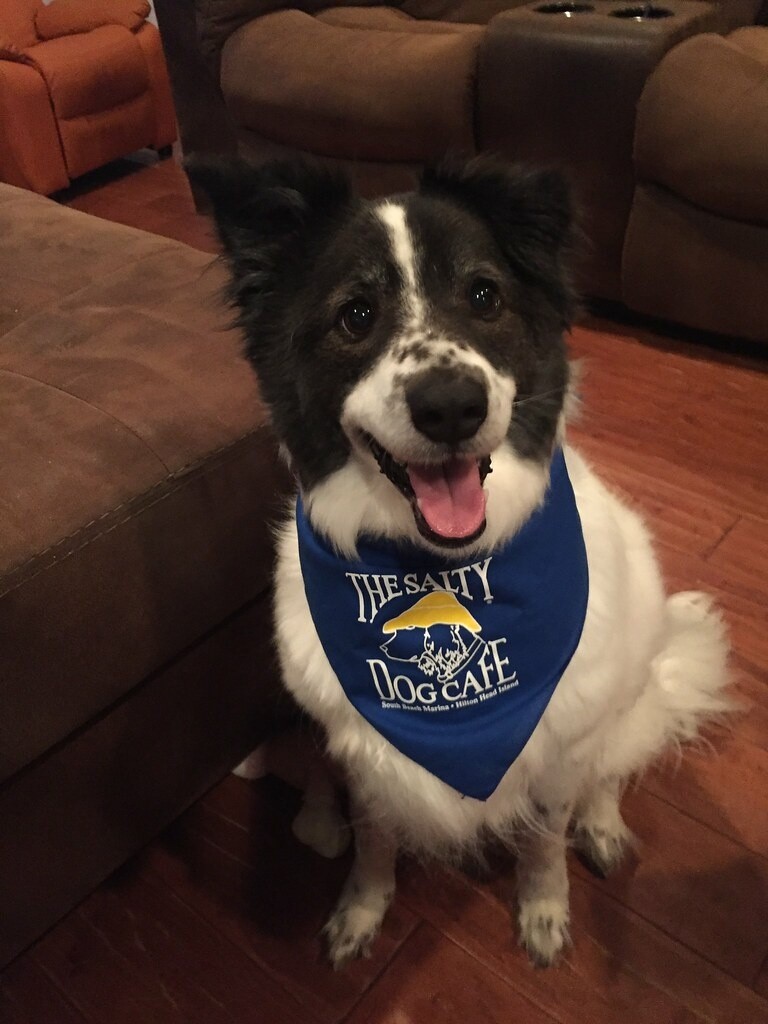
[180,151,748,968]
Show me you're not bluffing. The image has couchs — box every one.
[154,0,768,341]
[0,0,177,195]
[0,182,304,963]
